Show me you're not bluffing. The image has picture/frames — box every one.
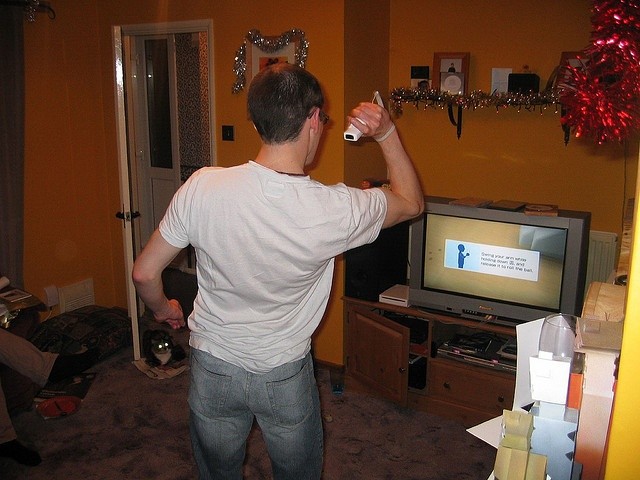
[431,52,469,100]
[250,37,299,75]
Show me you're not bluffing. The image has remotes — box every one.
[344,118,367,142]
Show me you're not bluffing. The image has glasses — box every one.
[309,107,330,125]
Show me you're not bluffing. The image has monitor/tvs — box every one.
[408,195,592,324]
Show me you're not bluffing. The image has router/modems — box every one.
[378,283,412,308]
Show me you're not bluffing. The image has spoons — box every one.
[53,402,67,417]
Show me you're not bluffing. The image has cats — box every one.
[142,329,176,370]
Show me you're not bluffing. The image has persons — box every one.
[447,63,455,72]
[132,62,425,480]
[0,327,101,467]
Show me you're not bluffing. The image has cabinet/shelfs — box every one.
[428,320,582,456]
[391,88,574,145]
[337,297,428,411]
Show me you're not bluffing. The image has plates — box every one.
[36,395,81,420]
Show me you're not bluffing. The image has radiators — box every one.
[583,229,617,296]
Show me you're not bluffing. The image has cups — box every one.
[538,315,584,374]
